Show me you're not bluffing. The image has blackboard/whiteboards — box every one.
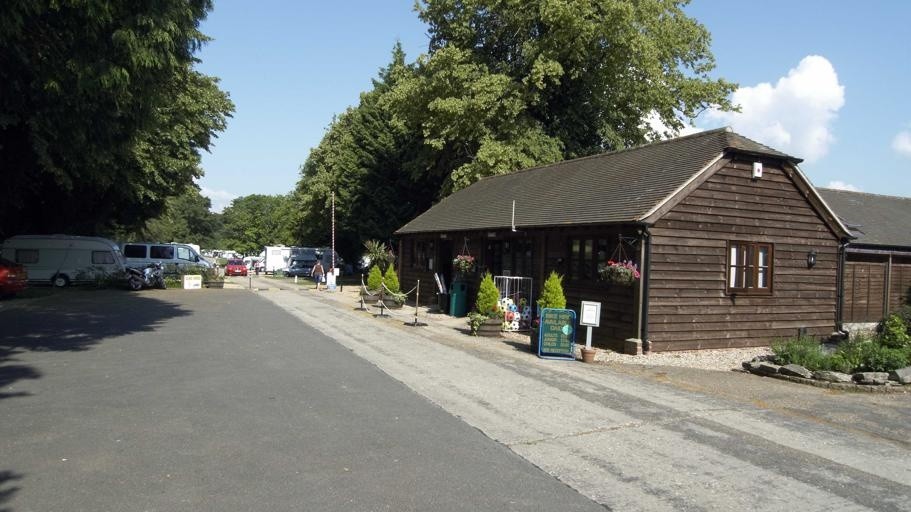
[539,308,576,355]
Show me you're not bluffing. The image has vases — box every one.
[580,348,597,362]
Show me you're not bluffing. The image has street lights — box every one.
[325,189,336,291]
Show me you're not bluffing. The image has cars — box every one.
[163,242,346,278]
[0,257,29,300]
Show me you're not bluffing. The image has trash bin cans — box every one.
[449,282,467,316]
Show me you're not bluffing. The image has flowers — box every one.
[597,259,642,286]
[452,254,477,274]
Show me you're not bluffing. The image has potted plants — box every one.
[377,263,407,308]
[465,271,505,338]
[361,264,384,305]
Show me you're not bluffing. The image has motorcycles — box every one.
[127,261,167,292]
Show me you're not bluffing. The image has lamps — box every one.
[807,249,817,267]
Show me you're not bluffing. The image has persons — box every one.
[311,260,325,289]
[253,260,261,275]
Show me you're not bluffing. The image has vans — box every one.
[120,241,212,283]
